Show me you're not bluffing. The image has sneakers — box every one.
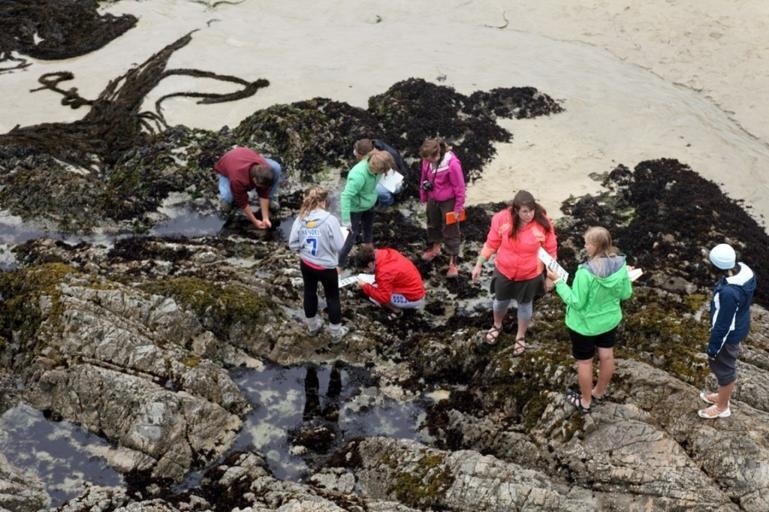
[331,325,349,344]
[377,310,404,323]
[700,392,730,406]
[306,319,324,337]
[698,403,730,418]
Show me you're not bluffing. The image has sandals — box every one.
[565,389,590,414]
[512,336,525,356]
[485,324,505,345]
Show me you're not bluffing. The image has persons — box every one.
[354,140,413,207]
[472,191,558,355]
[288,187,353,335]
[420,140,465,278]
[697,243,758,418]
[341,151,396,248]
[547,225,633,414]
[215,149,282,230]
[354,241,426,323]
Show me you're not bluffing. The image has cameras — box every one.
[422,180,433,192]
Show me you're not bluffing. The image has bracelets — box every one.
[477,256,486,264]
[553,278,561,284]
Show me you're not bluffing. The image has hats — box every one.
[709,243,736,270]
